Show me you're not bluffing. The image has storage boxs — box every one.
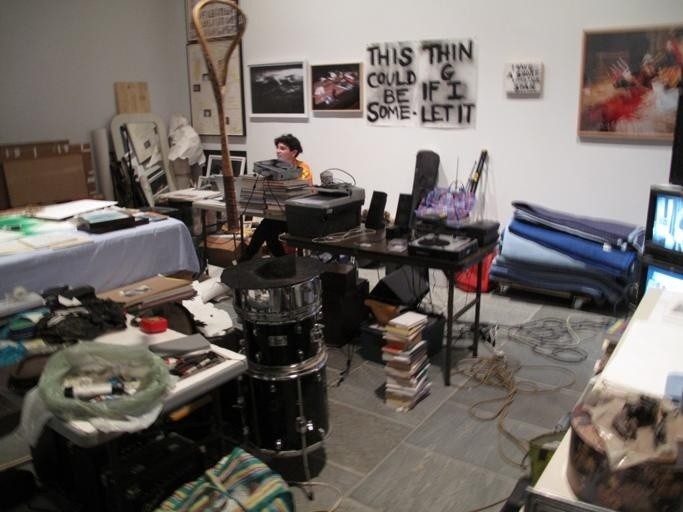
[31,325,248,512]
[198,235,241,269]
[361,308,446,365]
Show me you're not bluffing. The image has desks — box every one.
[278,222,499,387]
[0,198,201,323]
[523,273,683,512]
[157,189,286,268]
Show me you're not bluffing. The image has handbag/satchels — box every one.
[152,446,297,511]
[414,181,474,221]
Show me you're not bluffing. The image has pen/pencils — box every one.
[185,358,211,374]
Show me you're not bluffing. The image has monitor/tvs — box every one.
[205,154,247,177]
[639,183,683,274]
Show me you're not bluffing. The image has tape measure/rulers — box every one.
[138,316,168,333]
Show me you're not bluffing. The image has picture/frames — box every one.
[576,22,682,142]
[184,0,239,42]
[185,38,246,137]
[308,62,364,113]
[246,61,308,119]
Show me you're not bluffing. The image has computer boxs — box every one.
[321,263,360,348]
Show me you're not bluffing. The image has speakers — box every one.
[370,263,430,306]
[394,193,412,225]
[365,190,388,228]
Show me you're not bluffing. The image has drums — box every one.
[234,273,332,460]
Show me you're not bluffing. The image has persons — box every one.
[232,134,313,266]
[581,53,658,123]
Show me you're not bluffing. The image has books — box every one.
[381,311,432,413]
[238,175,315,216]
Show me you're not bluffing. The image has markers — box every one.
[64,381,124,399]
